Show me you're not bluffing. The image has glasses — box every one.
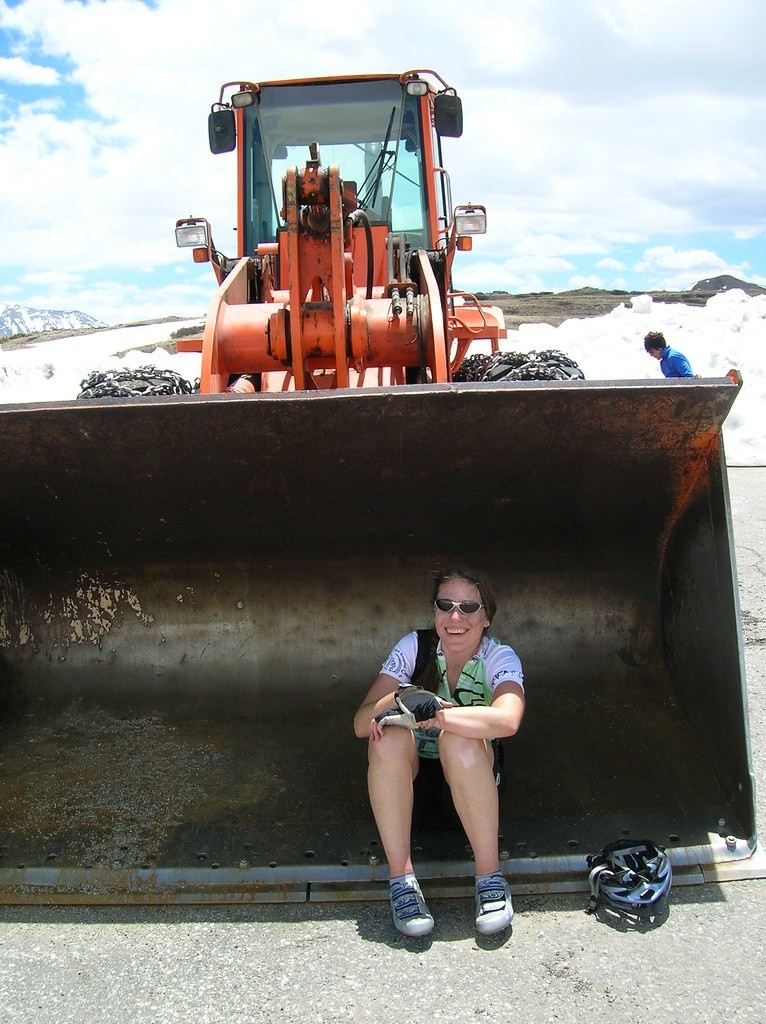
[433,598,485,615]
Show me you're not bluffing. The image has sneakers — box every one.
[474,869,514,936]
[388,872,435,937]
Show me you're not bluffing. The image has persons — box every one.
[644,331,692,377]
[355,566,525,937]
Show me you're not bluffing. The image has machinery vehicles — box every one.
[1,68,759,908]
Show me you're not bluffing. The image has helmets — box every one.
[585,839,672,915]
